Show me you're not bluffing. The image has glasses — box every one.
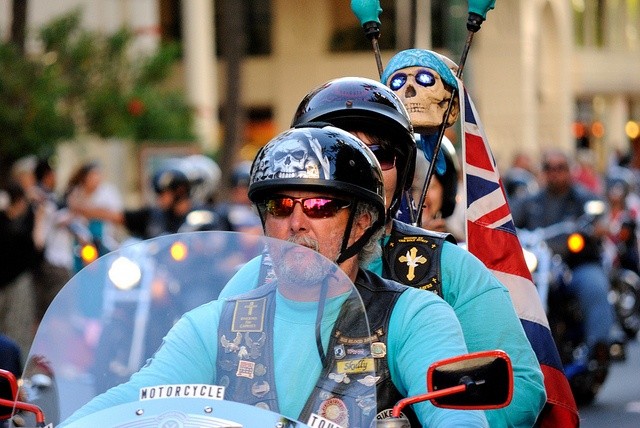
[263,192,353,218]
[369,144,399,171]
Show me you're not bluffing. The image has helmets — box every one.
[152,168,191,195]
[248,122,386,219]
[290,77,416,190]
[415,132,456,218]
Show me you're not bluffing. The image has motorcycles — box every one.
[517,218,637,409]
[7,228,515,428]
[60,208,230,395]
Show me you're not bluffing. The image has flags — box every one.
[456,82,583,428]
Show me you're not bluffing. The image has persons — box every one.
[409,130,461,235]
[589,178,635,282]
[32,156,59,268]
[224,160,262,236]
[53,121,490,428]
[69,151,246,311]
[497,131,612,370]
[1,140,45,346]
[570,135,606,199]
[507,154,538,194]
[59,160,108,362]
[608,155,640,187]
[288,76,547,428]
